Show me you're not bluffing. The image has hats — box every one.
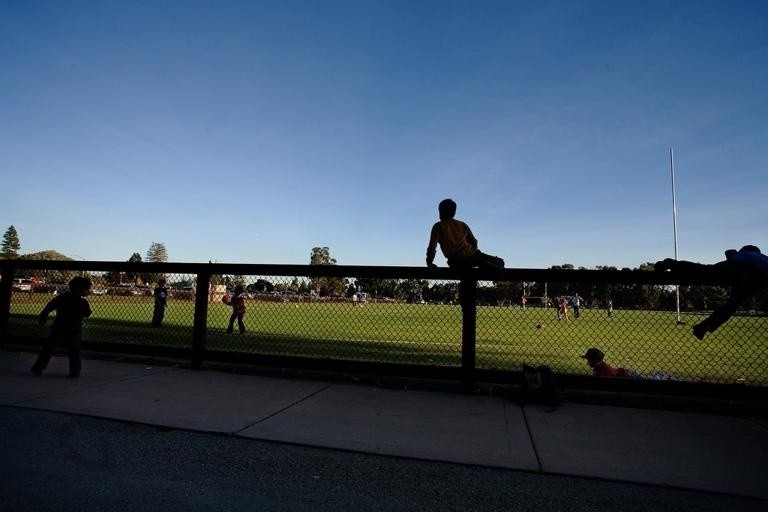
[579,348,605,360]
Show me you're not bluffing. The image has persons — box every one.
[653,244,768,342]
[221,284,247,335]
[150,280,169,326]
[580,347,675,382]
[554,292,584,320]
[29,275,95,376]
[607,299,614,317]
[426,197,503,268]
[352,292,358,306]
[519,296,527,310]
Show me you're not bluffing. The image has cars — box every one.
[13,277,172,296]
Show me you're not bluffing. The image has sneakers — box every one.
[66,371,80,378]
[27,367,48,377]
[693,323,707,340]
[654,258,675,273]
